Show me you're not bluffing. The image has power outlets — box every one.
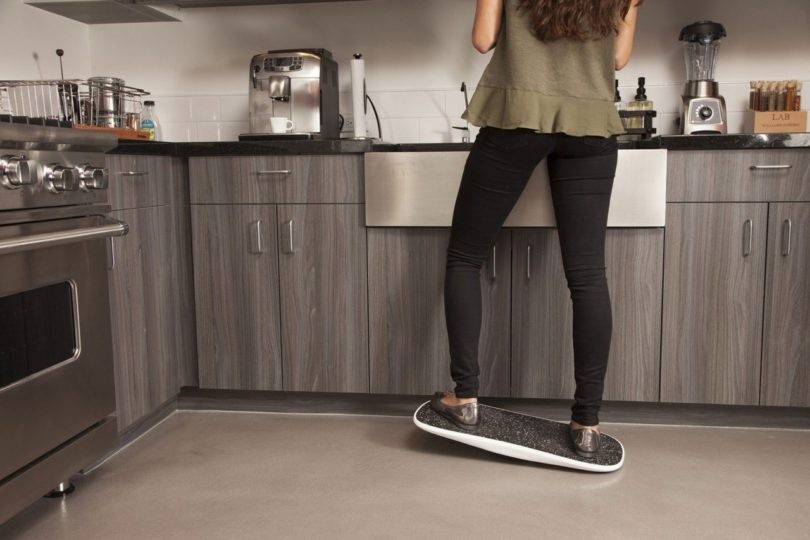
[338,113,353,132]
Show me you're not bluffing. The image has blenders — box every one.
[678,20,727,135]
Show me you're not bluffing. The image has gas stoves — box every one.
[0,121,119,209]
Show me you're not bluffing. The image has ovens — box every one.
[0,206,128,526]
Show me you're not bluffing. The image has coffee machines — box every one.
[238,47,340,140]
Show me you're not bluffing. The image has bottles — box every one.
[628,77,653,128]
[748,80,802,112]
[140,99,162,141]
[614,78,627,128]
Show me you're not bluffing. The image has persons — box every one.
[430,0,639,460]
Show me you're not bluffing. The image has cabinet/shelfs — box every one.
[104,148,810,442]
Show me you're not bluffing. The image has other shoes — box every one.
[569,421,601,458]
[432,391,480,431]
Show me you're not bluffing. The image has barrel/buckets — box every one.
[88,77,125,128]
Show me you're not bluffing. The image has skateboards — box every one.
[410,397,626,474]
